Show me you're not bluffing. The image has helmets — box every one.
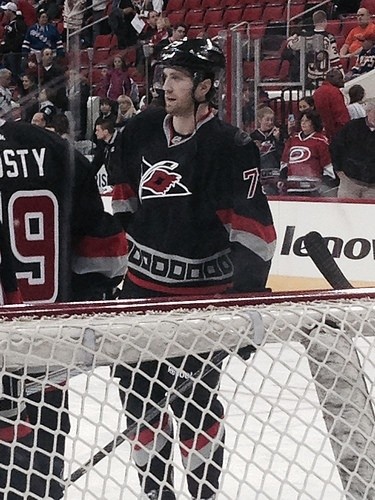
[154,38,226,89]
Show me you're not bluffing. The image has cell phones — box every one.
[288,113,294,126]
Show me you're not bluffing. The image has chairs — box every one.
[0,0,375,134]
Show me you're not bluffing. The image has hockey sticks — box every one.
[58,350,237,484]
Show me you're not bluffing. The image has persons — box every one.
[0,116,130,500]
[249,102,283,195]
[346,84,366,119]
[103,38,278,500]
[327,103,375,200]
[344,31,375,82]
[339,8,375,58]
[306,10,345,90]
[91,118,124,186]
[278,108,336,196]
[313,68,350,139]
[0,1,186,133]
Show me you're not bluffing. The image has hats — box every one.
[0,2,17,12]
